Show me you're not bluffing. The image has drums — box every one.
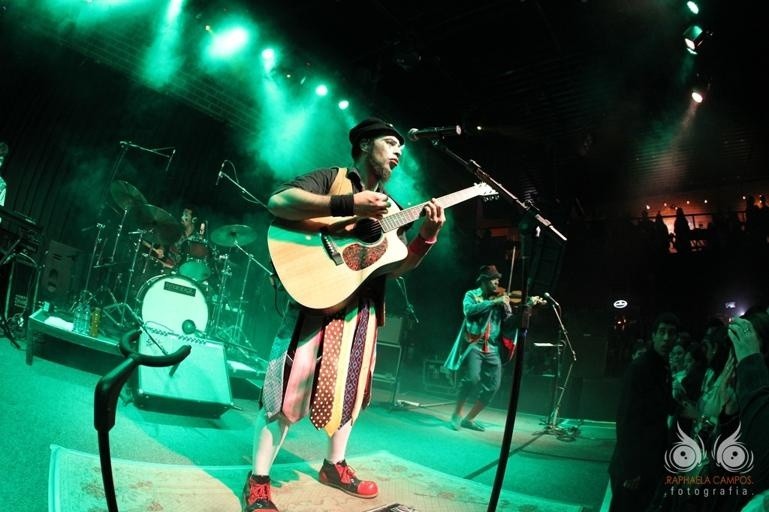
[177,235,220,282]
[133,271,212,338]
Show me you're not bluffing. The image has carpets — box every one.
[44,440,588,511]
[373,387,616,465]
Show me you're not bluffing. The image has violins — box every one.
[486,286,547,306]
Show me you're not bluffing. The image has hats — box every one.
[349,119,405,146]
[476,265,502,284]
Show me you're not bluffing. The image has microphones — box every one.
[407,124,462,142]
[165,150,176,172]
[544,292,559,306]
[215,163,224,185]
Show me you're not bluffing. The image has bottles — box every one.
[72,301,83,332]
[82,305,91,336]
[89,307,101,337]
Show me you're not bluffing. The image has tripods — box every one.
[67,224,258,354]
[533,307,577,436]
[379,277,409,413]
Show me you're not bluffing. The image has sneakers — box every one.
[242,472,280,511]
[318,459,379,498]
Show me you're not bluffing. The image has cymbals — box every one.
[211,224,257,248]
[108,176,182,244]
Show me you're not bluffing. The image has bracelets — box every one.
[329,194,354,217]
[409,233,437,257]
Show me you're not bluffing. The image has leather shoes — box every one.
[461,420,485,431]
[451,413,462,430]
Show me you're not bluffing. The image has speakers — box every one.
[132,326,233,418]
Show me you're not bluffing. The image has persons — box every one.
[157,207,219,288]
[609,313,769,512]
[242,117,446,512]
[444,265,514,432]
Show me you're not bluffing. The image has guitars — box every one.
[267,181,500,318]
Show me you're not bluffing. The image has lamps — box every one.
[681,0,717,108]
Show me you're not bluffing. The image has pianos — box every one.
[0,204,44,267]
